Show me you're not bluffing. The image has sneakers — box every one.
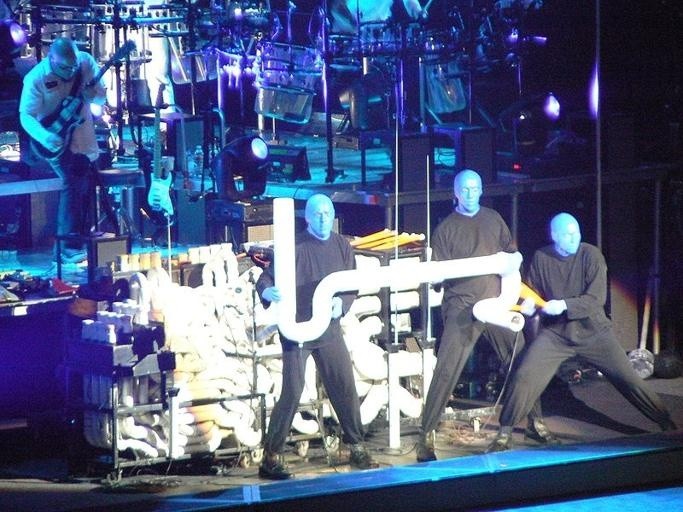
[415,428,437,461]
[482,431,512,454]
[258,458,295,481]
[348,442,379,470]
[51,248,88,266]
[524,415,561,447]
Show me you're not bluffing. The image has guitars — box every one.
[31,39,136,161]
[147,84,175,215]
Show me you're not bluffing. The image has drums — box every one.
[255,42,324,126]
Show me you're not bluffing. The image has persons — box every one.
[415,168,562,463]
[486,211,678,452]
[17,36,108,266]
[256,192,378,480]
